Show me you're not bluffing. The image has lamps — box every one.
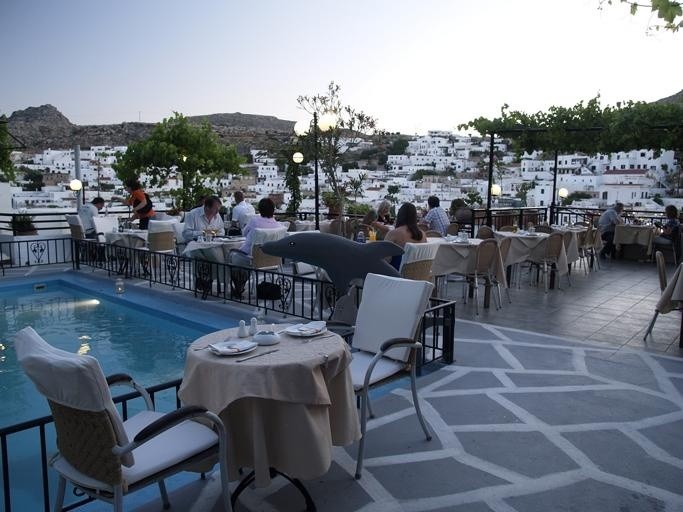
[559,188,568,206]
[491,184,502,206]
[70,178,82,198]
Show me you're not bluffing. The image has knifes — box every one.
[235,349,280,363]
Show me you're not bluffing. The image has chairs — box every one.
[603,221,682,351]
[350,273,437,478]
[14,327,229,509]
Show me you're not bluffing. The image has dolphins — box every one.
[258,232,409,300]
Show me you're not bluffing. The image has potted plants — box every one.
[6,211,40,236]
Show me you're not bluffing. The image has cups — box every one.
[445,230,471,245]
[222,213,231,222]
[513,220,593,236]
[112,216,140,234]
[198,224,247,242]
[355,231,376,243]
[625,217,654,226]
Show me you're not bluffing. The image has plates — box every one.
[211,317,329,356]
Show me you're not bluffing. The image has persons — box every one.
[366,198,395,240]
[225,191,254,237]
[228,200,282,298]
[597,203,625,260]
[637,205,680,263]
[110,178,156,230]
[383,203,428,273]
[417,195,449,237]
[182,196,225,295]
[77,197,106,262]
